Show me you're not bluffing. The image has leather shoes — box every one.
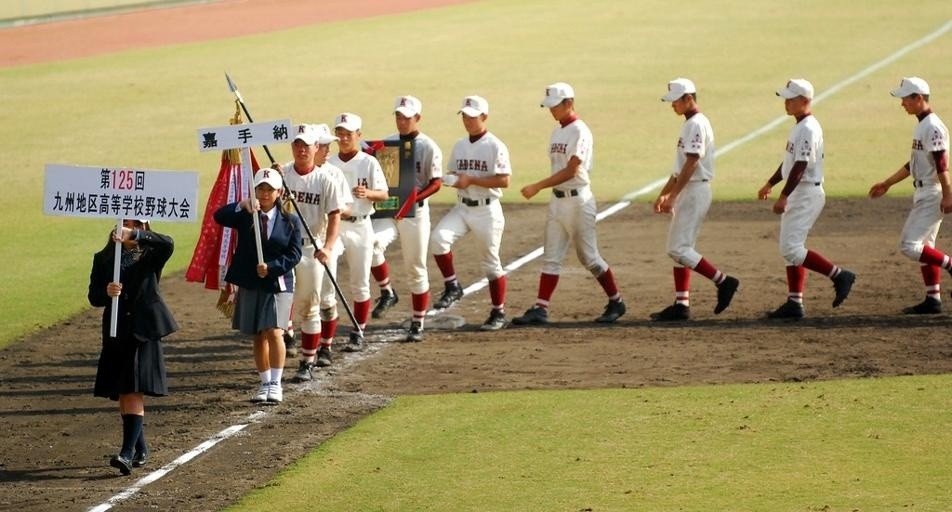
[109,455,132,476]
[132,451,148,468]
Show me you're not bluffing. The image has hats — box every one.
[314,124,340,144]
[539,82,575,108]
[889,76,930,98]
[290,124,318,146]
[775,78,814,100]
[660,78,696,103]
[254,168,282,189]
[456,95,489,118]
[331,113,361,131]
[393,95,422,119]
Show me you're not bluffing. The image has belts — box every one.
[912,179,940,188]
[457,194,498,207]
[301,238,317,247]
[552,184,591,199]
[686,179,711,182]
[797,182,823,187]
[340,215,368,223]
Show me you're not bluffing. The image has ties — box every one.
[260,214,268,242]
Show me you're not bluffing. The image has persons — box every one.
[212,168,303,403]
[282,122,354,366]
[758,78,856,320]
[512,82,627,326]
[430,94,512,332]
[649,77,739,322]
[88,219,181,476]
[868,76,952,314]
[371,95,443,343]
[270,123,347,383]
[326,113,390,353]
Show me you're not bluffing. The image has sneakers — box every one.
[714,275,739,315]
[432,282,464,310]
[594,298,626,323]
[295,360,315,382]
[832,265,856,309]
[406,320,424,342]
[903,295,944,315]
[316,349,332,367]
[649,303,690,320]
[766,299,806,322]
[267,380,282,401]
[344,329,365,352]
[479,309,506,331]
[511,306,547,325]
[254,382,269,402]
[285,333,298,358]
[371,288,400,320]
